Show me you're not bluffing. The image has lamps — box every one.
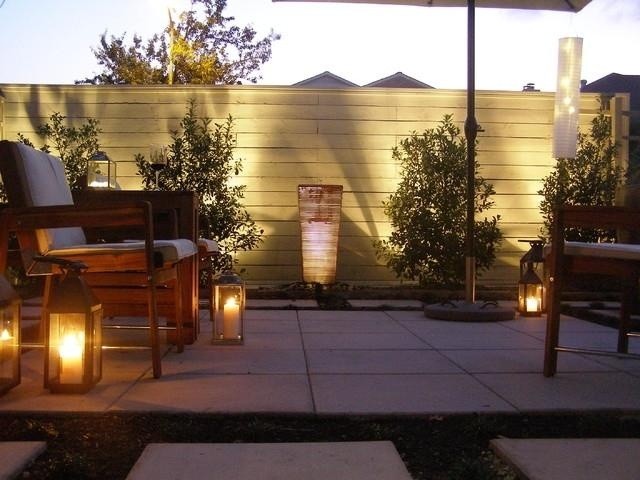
[1,273,23,395]
[291,183,344,294]
[210,267,246,346]
[85,150,117,190]
[517,238,545,318]
[30,247,106,394]
[556,33,584,203]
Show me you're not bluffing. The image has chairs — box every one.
[540,198,640,378]
[79,172,218,336]
[1,136,182,380]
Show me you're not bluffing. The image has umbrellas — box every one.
[272,0,591,304]
